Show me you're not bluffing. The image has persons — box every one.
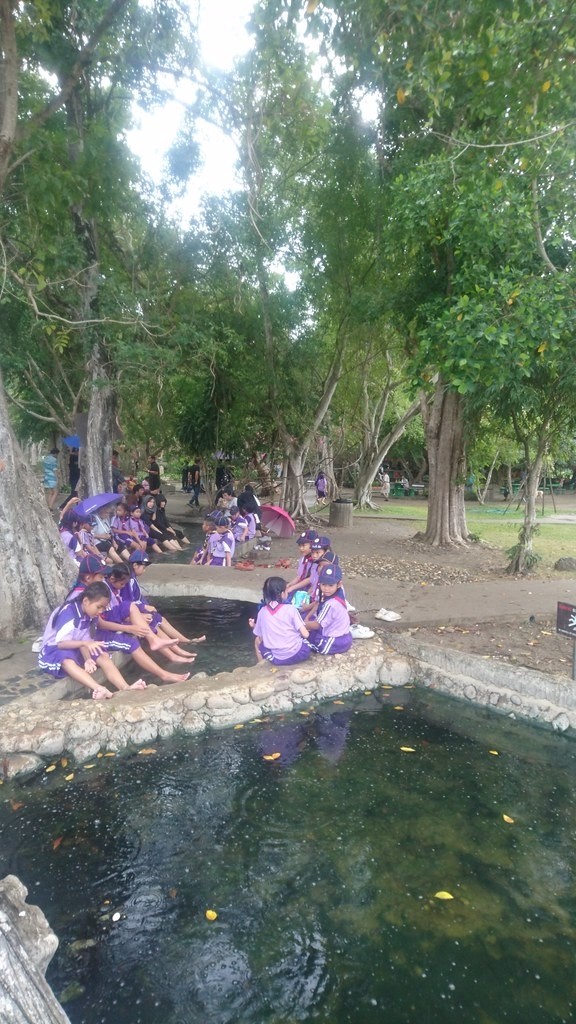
[187,457,203,508]
[144,456,161,494]
[38,549,206,700]
[379,470,390,502]
[215,456,234,488]
[401,475,409,488]
[190,483,260,568]
[283,529,354,655]
[58,476,192,568]
[56,447,81,512]
[112,448,125,493]
[314,470,328,505]
[182,460,191,494]
[44,447,61,512]
[248,576,310,665]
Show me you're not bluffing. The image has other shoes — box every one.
[57,507,62,512]
[231,532,290,571]
[375,608,402,621]
[346,599,355,611]
[31,636,44,652]
[349,624,375,639]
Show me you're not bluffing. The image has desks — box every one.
[394,482,406,496]
[504,484,521,493]
[547,484,560,494]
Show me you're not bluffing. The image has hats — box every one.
[215,517,230,527]
[313,551,339,566]
[129,504,140,512]
[79,555,113,574]
[207,510,222,518]
[309,536,330,549]
[129,549,152,565]
[319,564,342,584]
[228,506,239,515]
[296,530,319,544]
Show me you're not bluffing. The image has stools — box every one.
[556,488,566,495]
[390,489,396,496]
[540,487,550,494]
[397,487,405,496]
[500,487,505,494]
[408,487,416,496]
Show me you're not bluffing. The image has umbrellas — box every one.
[214,484,230,505]
[260,503,296,539]
[61,433,83,448]
[73,494,123,533]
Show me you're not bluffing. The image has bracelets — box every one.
[148,470,149,472]
[195,481,197,482]
